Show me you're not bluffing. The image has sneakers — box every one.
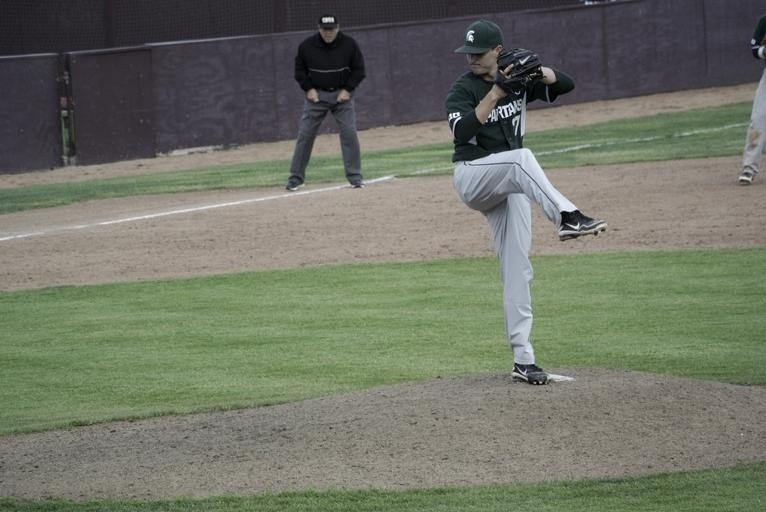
[350,177,365,188]
[558,210,609,242]
[510,363,549,386]
[287,178,305,191]
[738,168,758,184]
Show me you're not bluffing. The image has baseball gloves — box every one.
[495,48,542,96]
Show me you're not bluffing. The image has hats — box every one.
[454,20,503,54]
[318,14,339,28]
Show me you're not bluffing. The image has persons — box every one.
[286,14,367,191]
[738,15,766,185]
[444,19,608,386]
